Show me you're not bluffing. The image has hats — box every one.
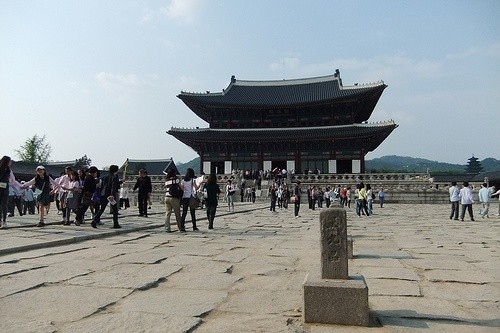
[36,165,45,171]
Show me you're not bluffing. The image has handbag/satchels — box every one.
[203,188,208,199]
[188,196,200,208]
[363,199,367,204]
[81,192,94,206]
[372,192,375,199]
[91,187,101,205]
[169,176,184,197]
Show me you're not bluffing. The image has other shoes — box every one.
[180,229,186,232]
[99,221,104,225]
[75,216,81,225]
[193,228,199,231]
[64,220,70,225]
[165,227,171,232]
[37,222,45,227]
[61,217,65,222]
[209,226,214,229]
[114,224,122,228]
[83,219,85,223]
[91,221,97,228]
[0,221,7,229]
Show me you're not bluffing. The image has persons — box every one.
[131,169,152,217]
[449,182,460,221]
[256,176,262,190]
[239,186,256,203]
[307,184,351,211]
[0,156,130,228]
[294,181,301,219]
[163,166,221,235]
[459,182,475,221]
[479,183,492,219]
[378,188,384,208]
[232,166,321,182]
[492,186,500,219]
[354,182,375,218]
[226,179,236,213]
[269,184,290,212]
